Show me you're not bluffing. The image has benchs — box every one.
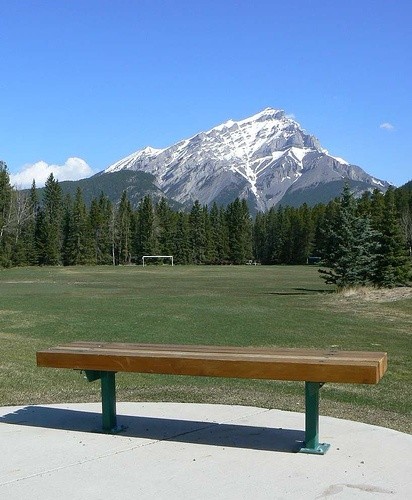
[34,338,388,454]
